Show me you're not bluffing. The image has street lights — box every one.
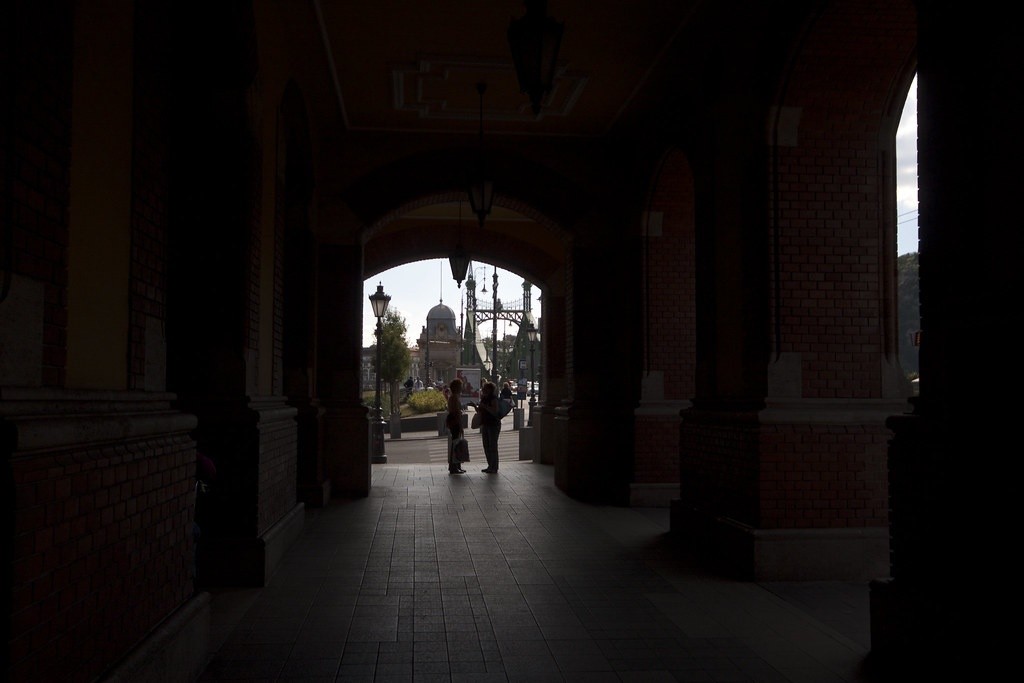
[368,279,393,463]
[415,315,430,393]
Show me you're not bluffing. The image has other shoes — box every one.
[459,468,466,472]
[481,468,497,474]
[450,469,464,474]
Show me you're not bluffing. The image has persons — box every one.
[414,376,423,390]
[432,380,436,387]
[446,379,468,475]
[466,382,503,474]
[502,380,518,403]
[442,384,449,399]
[404,376,414,397]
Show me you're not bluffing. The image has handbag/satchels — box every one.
[453,430,471,463]
[471,413,481,430]
[497,399,512,420]
